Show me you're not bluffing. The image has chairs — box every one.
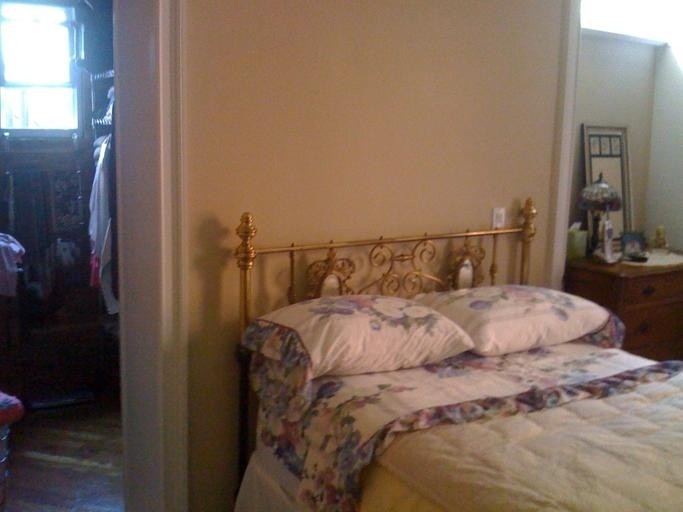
[1,131,118,420]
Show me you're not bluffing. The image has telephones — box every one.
[593,204,623,264]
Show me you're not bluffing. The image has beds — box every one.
[227,197,683,511]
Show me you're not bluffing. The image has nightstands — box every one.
[564,245,683,362]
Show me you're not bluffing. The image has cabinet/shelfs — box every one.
[89,67,114,144]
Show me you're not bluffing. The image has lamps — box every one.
[575,171,622,259]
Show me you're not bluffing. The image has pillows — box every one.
[407,282,628,358]
[239,294,476,424]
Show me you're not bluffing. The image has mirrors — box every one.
[581,119,636,251]
